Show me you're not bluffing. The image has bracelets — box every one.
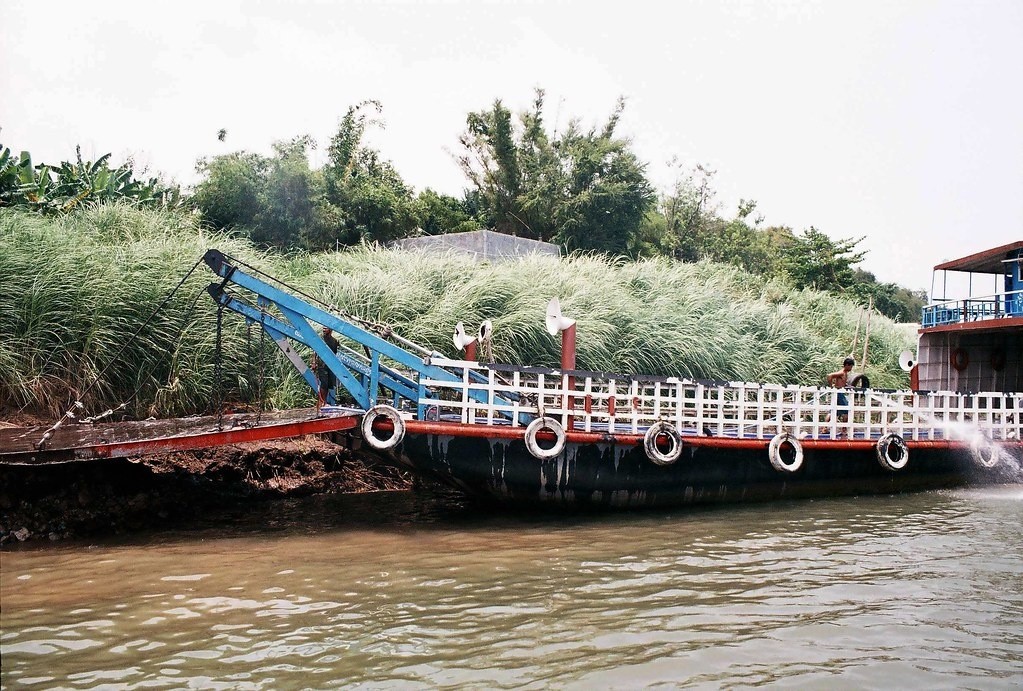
[312,362,316,364]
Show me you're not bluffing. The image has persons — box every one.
[311,325,339,419]
[822,358,857,436]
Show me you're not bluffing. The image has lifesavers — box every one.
[359,403,405,451]
[846,374,870,399]
[768,431,804,473]
[970,436,997,467]
[876,433,909,471]
[643,421,683,465]
[524,416,568,461]
